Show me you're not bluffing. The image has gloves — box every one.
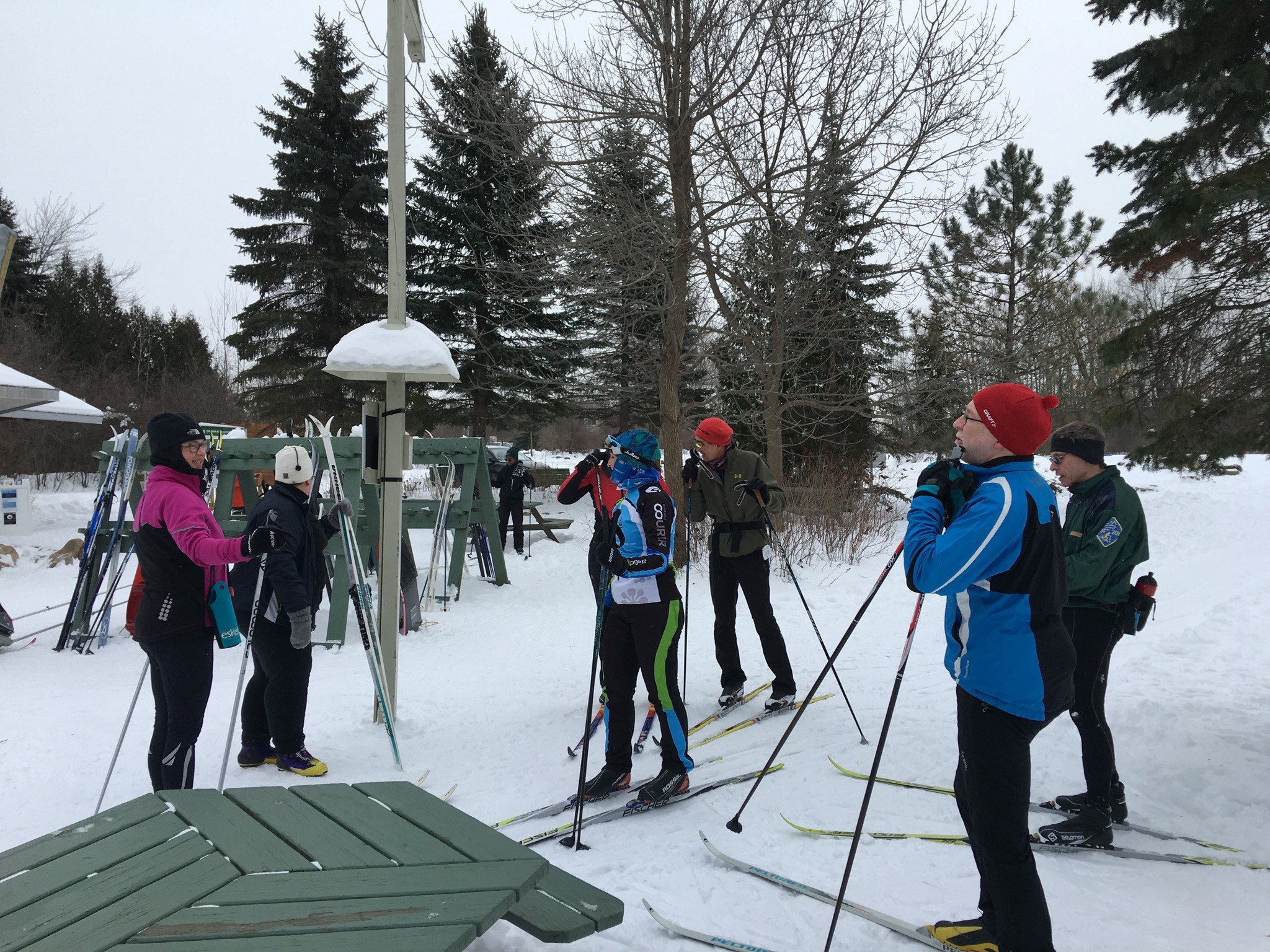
[917,462,974,525]
[682,458,698,483]
[287,606,312,649]
[241,525,286,558]
[528,474,534,483]
[582,448,609,468]
[594,541,627,576]
[744,477,770,505]
[328,498,354,530]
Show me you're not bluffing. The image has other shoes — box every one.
[502,546,505,551]
[516,547,524,552]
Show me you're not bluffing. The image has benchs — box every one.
[502,518,574,531]
[528,466,572,491]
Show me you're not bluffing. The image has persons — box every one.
[903,382,1077,952]
[133,411,286,794]
[681,418,796,712]
[229,446,354,777]
[494,445,535,554]
[1038,421,1150,846]
[555,430,695,802]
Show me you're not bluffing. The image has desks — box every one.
[496,500,561,543]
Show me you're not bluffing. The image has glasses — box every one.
[181,441,210,453]
[504,458,512,461]
[603,435,622,455]
[963,413,982,424]
[694,440,708,448]
[1049,453,1071,464]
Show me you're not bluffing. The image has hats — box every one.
[611,430,661,469]
[695,418,733,446]
[973,383,1059,456]
[505,445,521,460]
[274,445,314,484]
[147,412,206,478]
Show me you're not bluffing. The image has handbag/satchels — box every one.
[1125,589,1155,635]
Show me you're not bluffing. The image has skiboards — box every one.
[640,830,967,952]
[286,417,342,437]
[425,430,491,578]
[0,637,36,654]
[687,685,837,748]
[308,414,405,772]
[566,702,656,758]
[414,769,459,800]
[202,430,222,505]
[488,755,783,842]
[782,758,1268,868]
[424,455,456,612]
[298,439,318,504]
[51,425,148,652]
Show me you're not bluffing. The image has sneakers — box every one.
[932,781,1129,952]
[585,682,798,803]
[240,746,328,776]
